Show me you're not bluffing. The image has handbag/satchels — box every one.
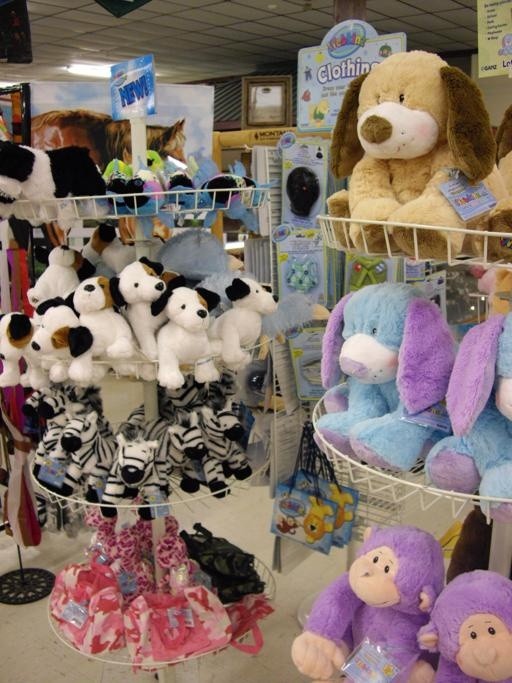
[271,470,359,554]
[49,524,275,672]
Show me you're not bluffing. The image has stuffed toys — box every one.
[290,522,512,683]
[286,51,511,683]
[0,139,280,505]
[325,49,512,263]
[315,281,512,520]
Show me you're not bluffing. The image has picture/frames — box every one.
[240,75,293,129]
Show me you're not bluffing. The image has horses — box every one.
[29,108,188,252]
